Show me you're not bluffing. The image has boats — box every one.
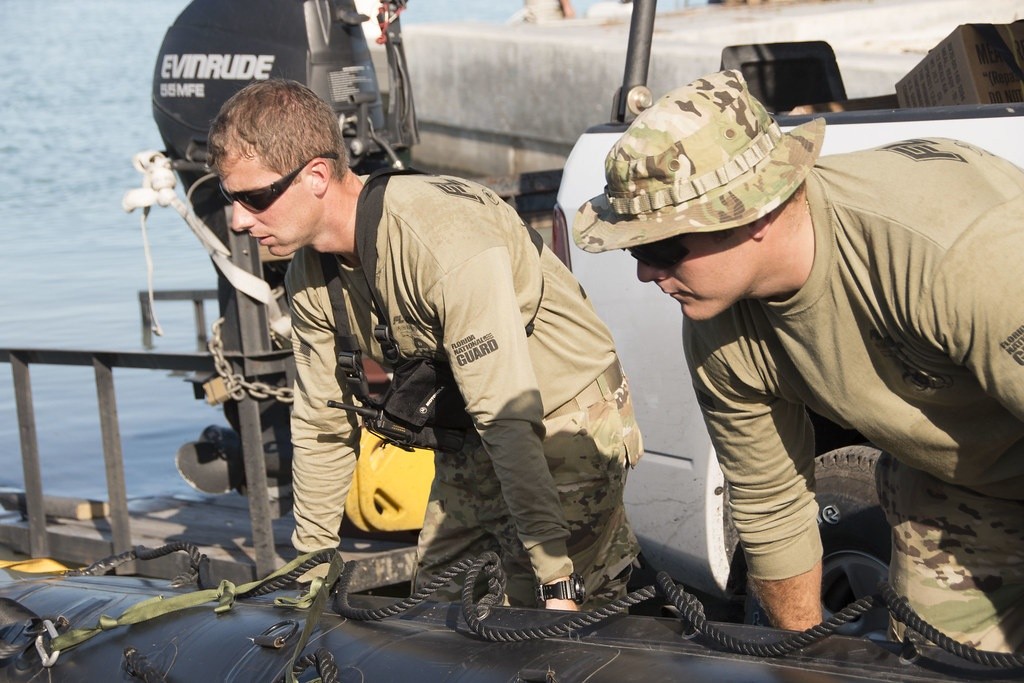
[147,0,1024,234]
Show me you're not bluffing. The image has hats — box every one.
[572,69,827,254]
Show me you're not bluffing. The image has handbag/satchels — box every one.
[361,356,474,454]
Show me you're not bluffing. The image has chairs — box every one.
[720,40,847,113]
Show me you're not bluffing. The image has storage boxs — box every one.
[895,24,1024,108]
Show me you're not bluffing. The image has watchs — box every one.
[534,572,587,607]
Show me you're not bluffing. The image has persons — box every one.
[203,76,645,613]
[572,67,1023,652]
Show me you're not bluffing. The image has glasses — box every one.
[631,238,689,269]
[219,152,339,214]
